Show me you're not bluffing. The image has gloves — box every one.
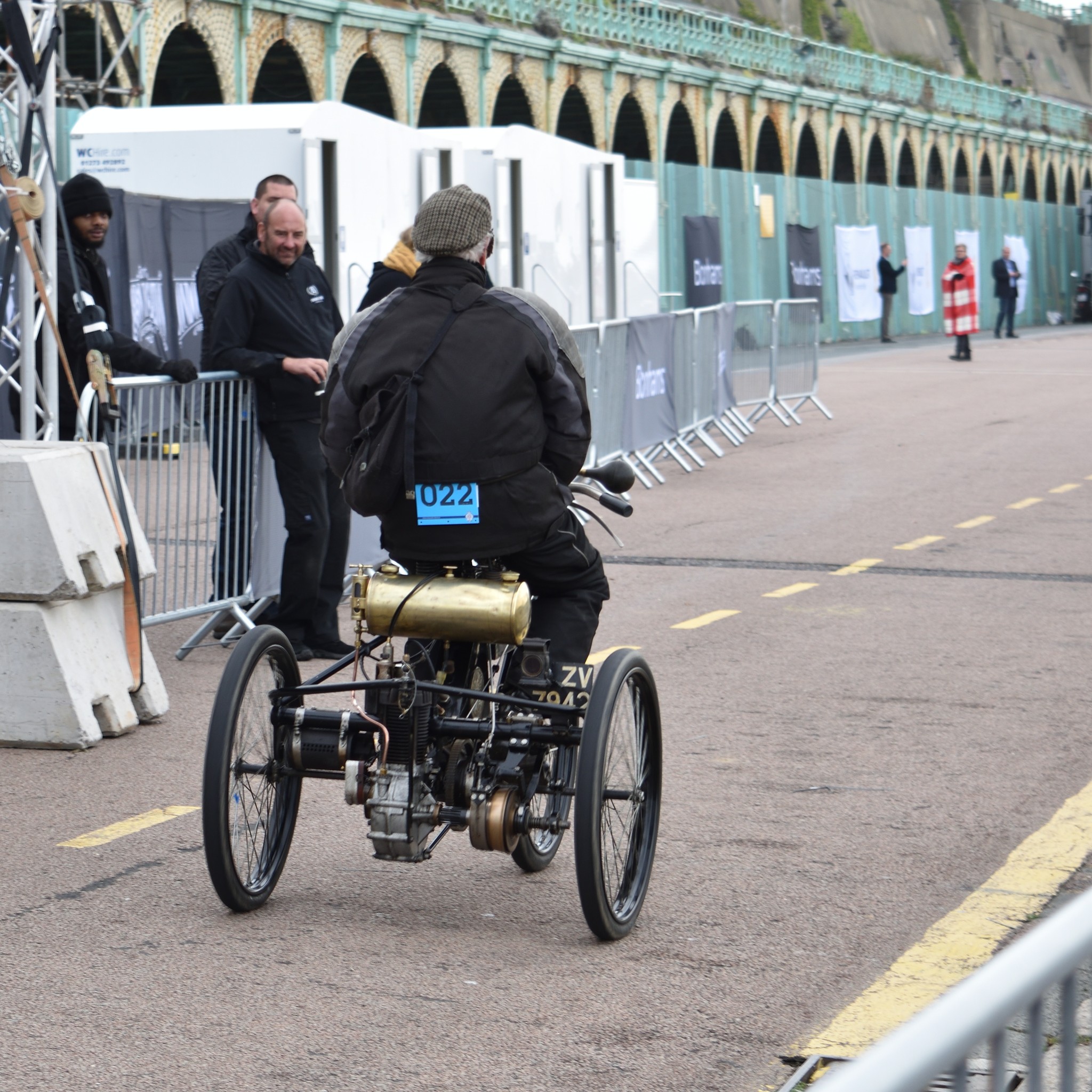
[159,357,198,384]
[954,273,963,280]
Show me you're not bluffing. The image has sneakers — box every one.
[314,639,358,660]
[269,636,312,663]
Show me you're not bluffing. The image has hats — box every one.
[412,184,492,256]
[60,173,113,220]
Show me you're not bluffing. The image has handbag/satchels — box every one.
[340,367,424,517]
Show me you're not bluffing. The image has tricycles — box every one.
[199,459,666,939]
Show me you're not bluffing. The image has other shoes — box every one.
[882,338,895,343]
[994,332,1018,338]
[212,606,252,638]
[948,353,971,360]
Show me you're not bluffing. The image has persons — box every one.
[314,183,611,768]
[940,243,981,362]
[6,172,200,444]
[876,242,909,344]
[989,246,1021,339]
[357,224,496,314]
[195,172,318,639]
[202,197,357,661]
